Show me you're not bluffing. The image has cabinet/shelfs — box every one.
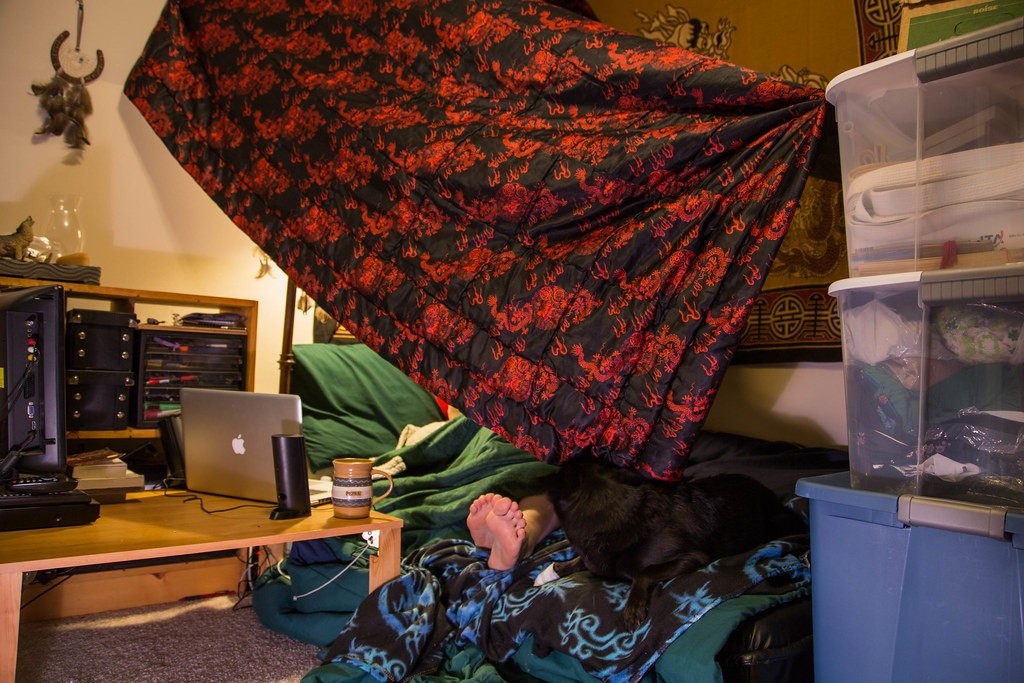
[0,274,258,476]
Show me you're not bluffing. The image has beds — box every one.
[250,422,849,683]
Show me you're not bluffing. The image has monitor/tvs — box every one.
[0,283,68,478]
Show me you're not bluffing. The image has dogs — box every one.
[533,441,810,635]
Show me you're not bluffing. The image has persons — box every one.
[467,488,561,571]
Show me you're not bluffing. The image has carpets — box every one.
[15,592,323,683]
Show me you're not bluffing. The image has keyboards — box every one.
[0,487,92,507]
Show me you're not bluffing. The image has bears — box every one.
[0,216,35,260]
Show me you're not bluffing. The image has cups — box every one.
[331,457,394,519]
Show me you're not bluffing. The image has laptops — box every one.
[180,387,335,506]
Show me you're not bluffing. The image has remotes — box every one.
[6,478,78,493]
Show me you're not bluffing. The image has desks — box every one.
[0,485,404,683]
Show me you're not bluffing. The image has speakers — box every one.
[159,414,188,490]
[269,434,312,520]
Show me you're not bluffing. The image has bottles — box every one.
[41,192,85,261]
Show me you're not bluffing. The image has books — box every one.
[68,445,144,490]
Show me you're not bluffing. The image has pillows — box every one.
[290,342,448,474]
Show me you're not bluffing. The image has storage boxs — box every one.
[825,15,1023,278]
[794,471,1023,682]
[824,261,1024,510]
